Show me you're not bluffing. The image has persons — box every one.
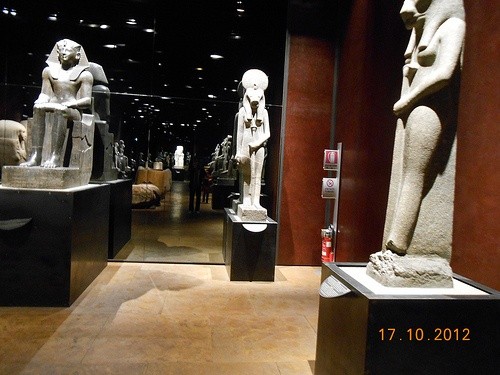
[201,169,211,204]
[114,138,191,179]
[186,154,204,215]
[211,134,232,173]
[22,38,91,169]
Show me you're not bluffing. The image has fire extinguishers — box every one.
[320,228,334,264]
